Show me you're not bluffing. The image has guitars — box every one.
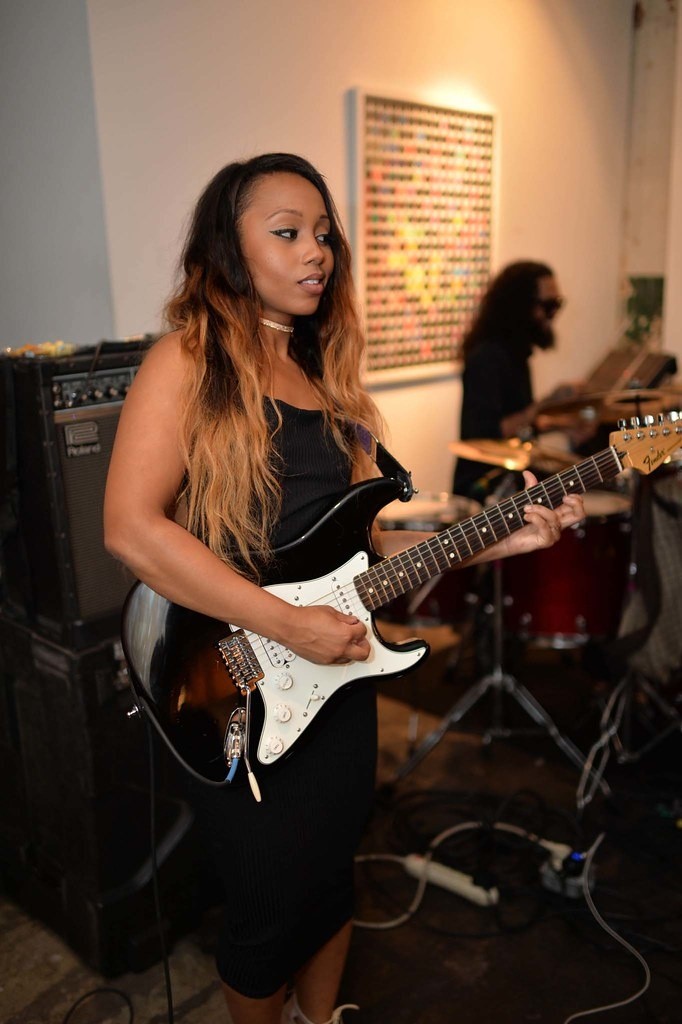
[121,411,682,787]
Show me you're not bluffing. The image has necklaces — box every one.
[255,316,296,335]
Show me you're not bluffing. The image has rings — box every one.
[549,522,561,534]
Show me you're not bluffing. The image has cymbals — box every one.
[447,436,587,480]
[538,386,682,426]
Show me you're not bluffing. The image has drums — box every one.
[478,491,633,650]
[368,490,486,624]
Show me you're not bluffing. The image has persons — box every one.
[100,151,588,1023]
[448,257,564,604]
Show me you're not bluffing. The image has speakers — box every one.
[0,335,199,979]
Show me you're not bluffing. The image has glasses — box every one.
[537,296,562,319]
